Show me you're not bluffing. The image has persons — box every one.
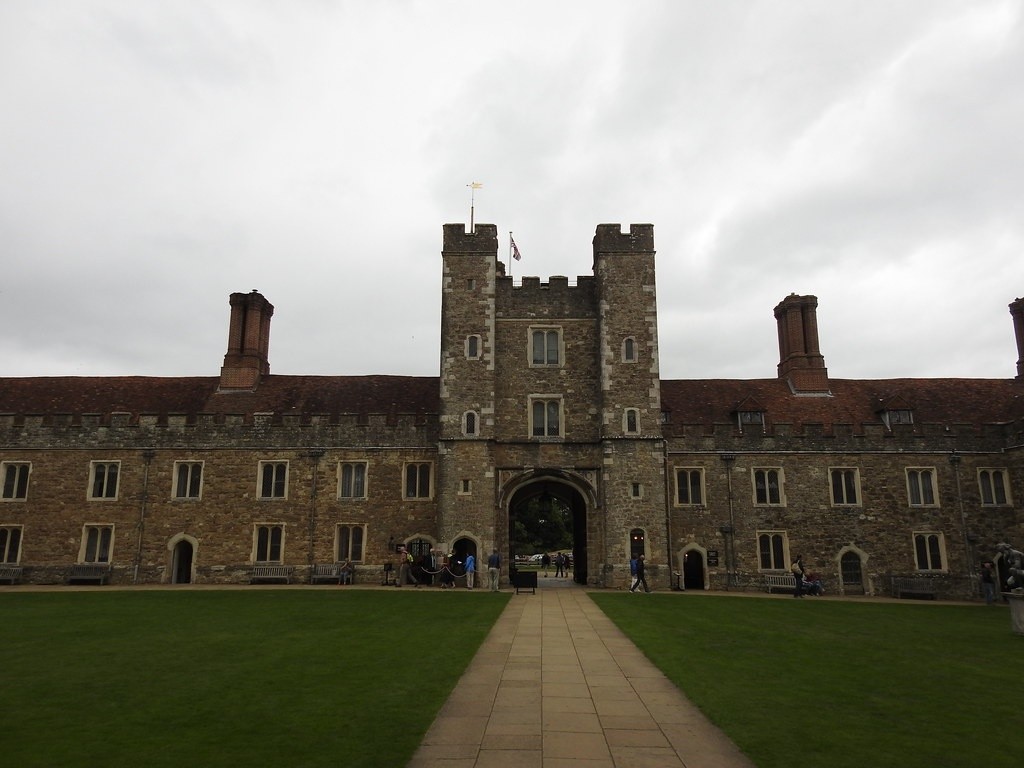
[487,548,500,592]
[396,547,419,588]
[424,548,475,589]
[997,543,1024,590]
[338,557,351,584]
[541,551,551,577]
[555,552,570,578]
[629,553,651,593]
[982,563,995,604]
[792,554,825,598]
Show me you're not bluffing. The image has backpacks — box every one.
[403,551,413,563]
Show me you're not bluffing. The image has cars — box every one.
[528,552,573,562]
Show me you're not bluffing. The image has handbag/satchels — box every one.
[791,560,802,575]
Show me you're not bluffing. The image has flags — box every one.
[511,237,522,261]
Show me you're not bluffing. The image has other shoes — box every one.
[645,590,651,593]
[630,590,633,593]
[636,589,641,592]
[394,585,402,587]
[415,581,419,587]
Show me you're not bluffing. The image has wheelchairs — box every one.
[802,580,825,597]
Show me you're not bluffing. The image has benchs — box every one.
[247,566,294,584]
[311,564,353,583]
[765,574,797,594]
[64,564,110,586]
[0,567,22,586]
[891,578,939,601]
